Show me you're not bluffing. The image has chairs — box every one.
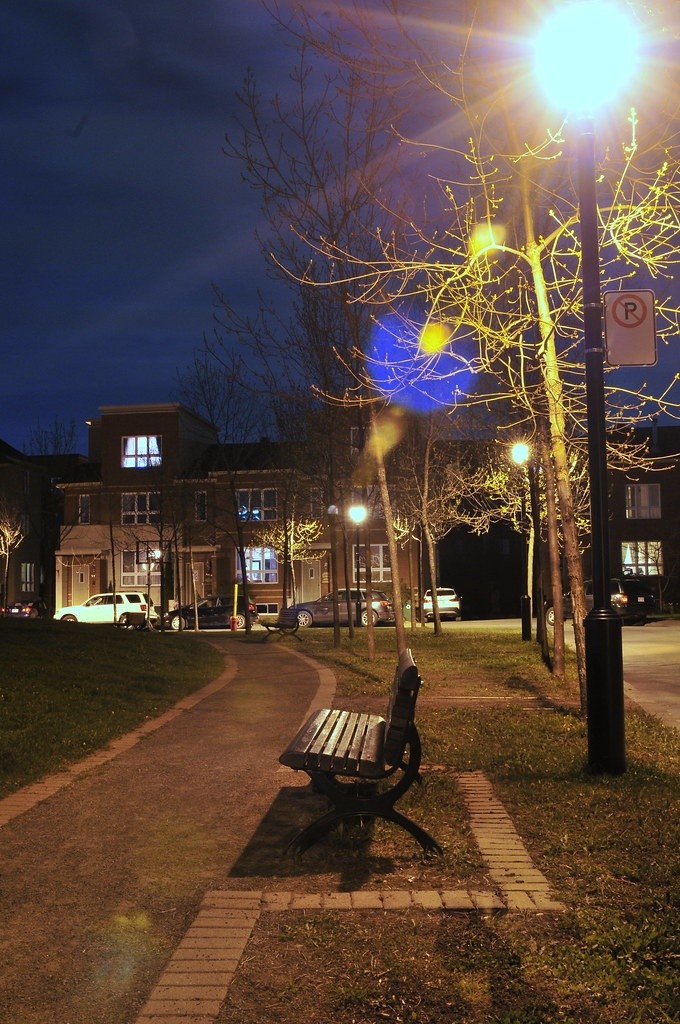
[342,593,346,599]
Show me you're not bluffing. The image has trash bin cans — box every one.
[402,600,411,622]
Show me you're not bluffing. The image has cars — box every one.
[53,592,148,631]
[2,600,48,618]
[162,596,259,630]
[546,578,646,626]
[288,587,462,628]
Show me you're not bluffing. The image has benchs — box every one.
[261,608,302,643]
[278,648,444,859]
[112,612,147,631]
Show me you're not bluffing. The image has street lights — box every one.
[350,508,365,627]
[528,0,644,774]
[143,551,161,628]
